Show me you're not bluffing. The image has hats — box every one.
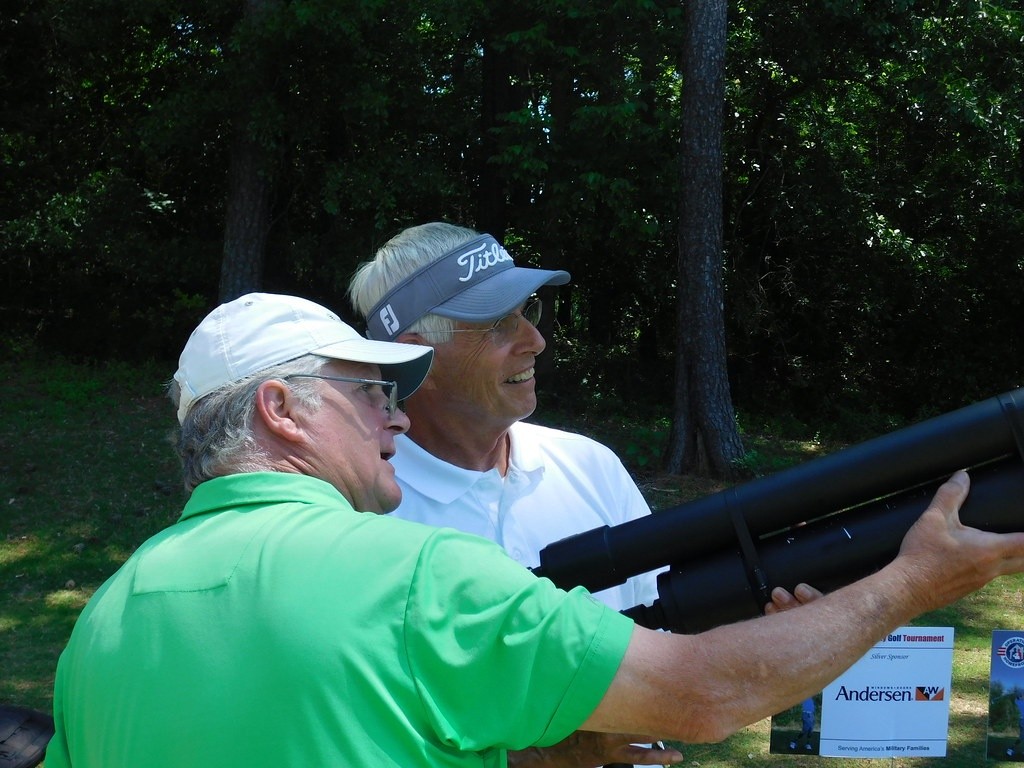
[176,292,434,426]
[366,233,571,342]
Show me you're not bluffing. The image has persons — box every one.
[789,697,815,750]
[1006,689,1024,756]
[350,221,825,768]
[43,293,1024,768]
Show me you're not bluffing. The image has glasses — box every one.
[402,296,543,349]
[285,373,398,420]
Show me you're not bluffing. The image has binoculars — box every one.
[524,385,1024,636]
